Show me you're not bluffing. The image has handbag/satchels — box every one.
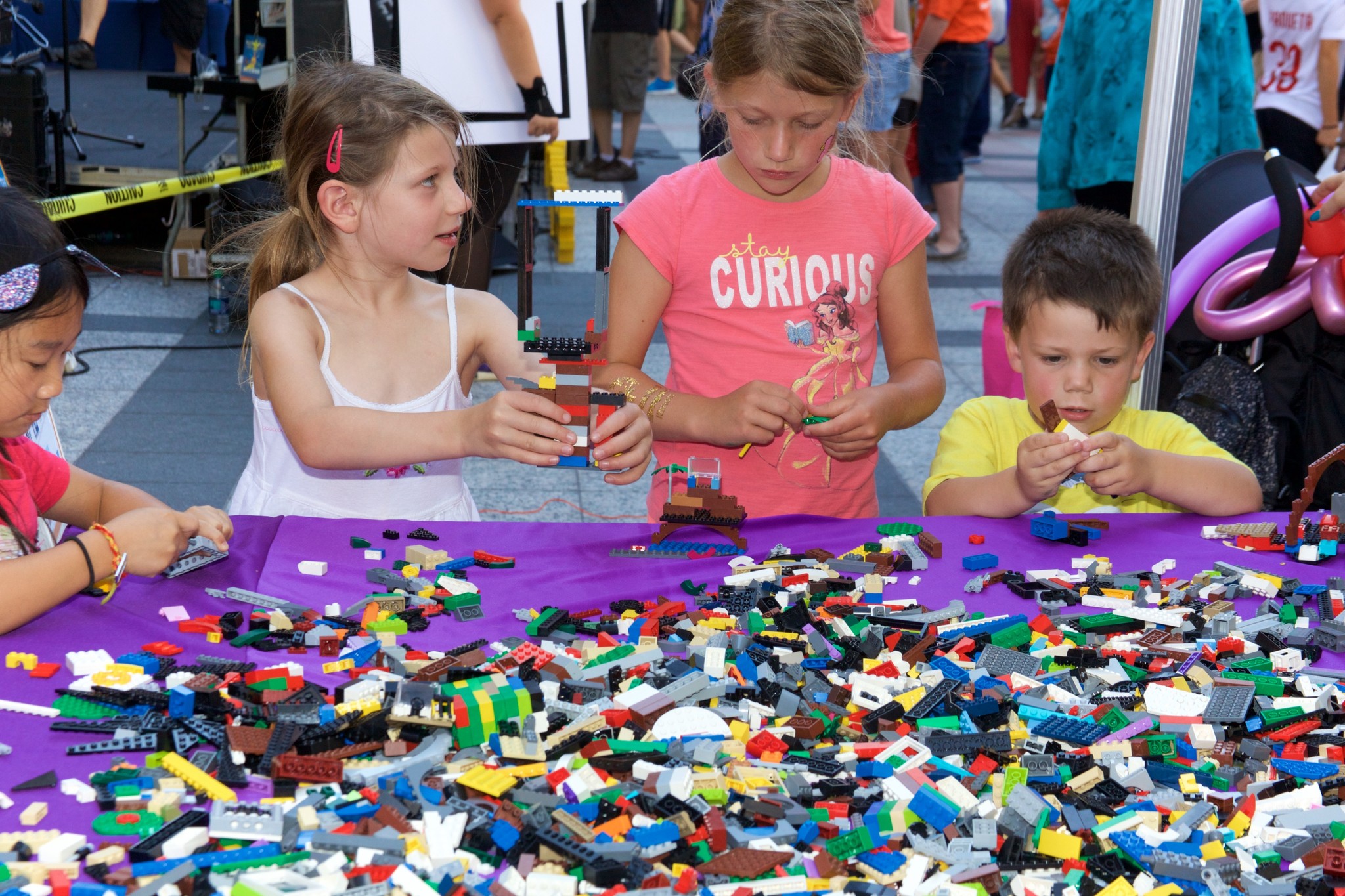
[678,14,713,99]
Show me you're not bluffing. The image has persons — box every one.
[0,0,1084,288]
[1035,0,1345,222]
[0,187,235,634]
[923,206,1261,515]
[227,64,653,521]
[589,0,944,523]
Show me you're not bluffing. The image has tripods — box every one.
[43,1,145,161]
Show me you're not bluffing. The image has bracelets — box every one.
[1321,124,1338,130]
[86,522,128,606]
[64,535,95,590]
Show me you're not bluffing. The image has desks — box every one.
[144,65,295,289]
[0,511,1345,896]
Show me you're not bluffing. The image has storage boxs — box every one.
[167,225,214,283]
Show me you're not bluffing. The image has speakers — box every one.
[0,59,48,199]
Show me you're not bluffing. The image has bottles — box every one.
[208,269,229,335]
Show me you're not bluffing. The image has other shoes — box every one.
[646,77,677,95]
[53,42,97,70]
[925,231,969,259]
[1000,92,1028,128]
[575,155,638,181]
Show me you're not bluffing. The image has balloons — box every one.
[1163,186,1345,341]
